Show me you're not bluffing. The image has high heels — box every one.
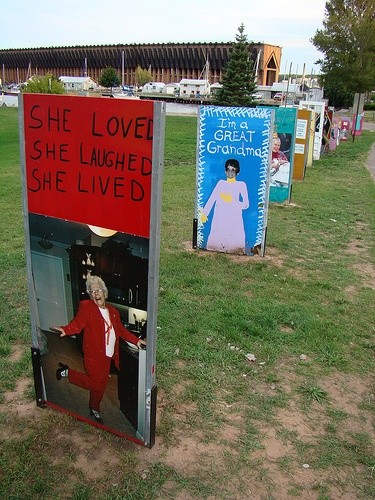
[56,362,70,381]
[90,409,104,424]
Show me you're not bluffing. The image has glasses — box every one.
[226,168,237,173]
[89,289,104,294]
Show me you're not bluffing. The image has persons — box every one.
[48,274,146,422]
[224,159,240,178]
[271,136,290,170]
